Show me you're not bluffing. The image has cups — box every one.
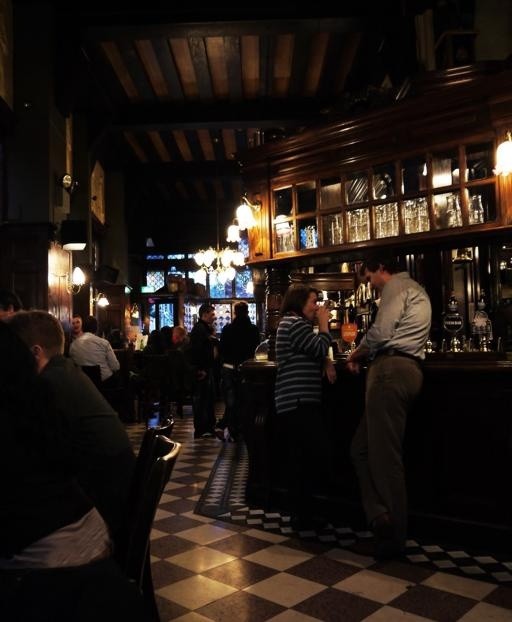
[326,195,463,245]
[470,195,484,225]
[315,307,332,321]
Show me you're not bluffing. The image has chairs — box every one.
[34,414,181,620]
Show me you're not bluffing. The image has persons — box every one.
[0,311,138,529]
[274,286,346,531]
[346,247,432,558]
[69,313,120,385]
[143,302,261,441]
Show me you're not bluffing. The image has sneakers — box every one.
[350,510,408,556]
[197,426,229,442]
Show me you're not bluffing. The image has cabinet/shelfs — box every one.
[229,118,511,353]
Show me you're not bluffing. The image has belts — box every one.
[378,347,425,368]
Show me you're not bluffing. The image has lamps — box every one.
[65,266,85,296]
[191,128,246,289]
[53,217,88,253]
